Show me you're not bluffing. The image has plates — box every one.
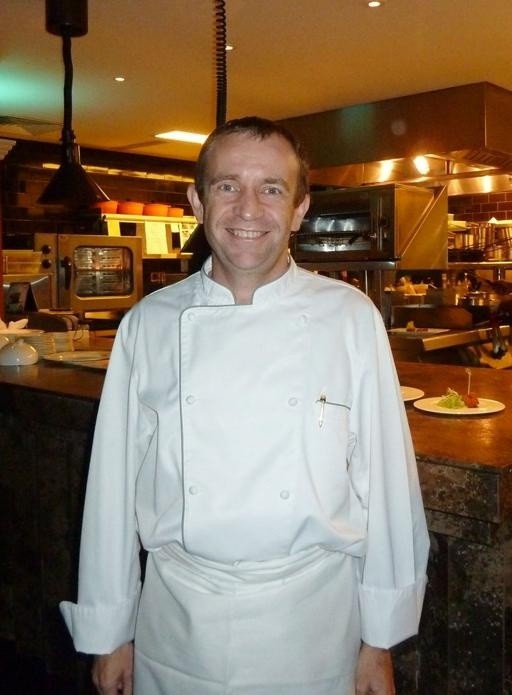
[0,328,91,355]
[42,350,114,362]
[410,394,504,418]
[399,385,425,403]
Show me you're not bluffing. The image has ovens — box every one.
[284,178,437,266]
[30,230,147,313]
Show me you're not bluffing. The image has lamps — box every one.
[35,0,110,204]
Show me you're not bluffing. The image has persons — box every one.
[59,116,430,695]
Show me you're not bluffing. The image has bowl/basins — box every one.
[90,199,186,218]
[0,341,41,367]
